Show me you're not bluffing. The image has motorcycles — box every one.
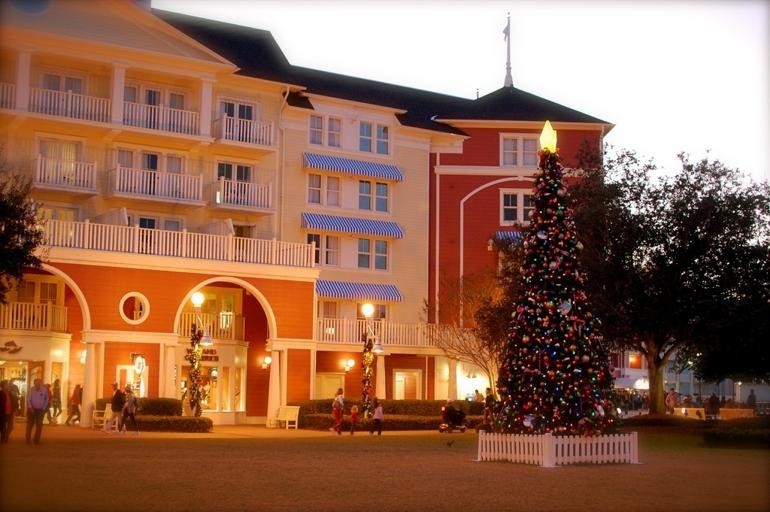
[439,409,466,432]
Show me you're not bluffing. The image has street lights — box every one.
[189,292,204,418]
[362,304,374,418]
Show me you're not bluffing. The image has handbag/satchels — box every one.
[332,401,341,407]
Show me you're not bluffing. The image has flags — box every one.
[503,25,508,40]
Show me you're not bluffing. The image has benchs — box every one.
[92,403,118,429]
[265,405,301,430]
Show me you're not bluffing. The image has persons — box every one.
[484,388,496,424]
[614,388,757,420]
[350,405,359,435]
[445,399,457,426]
[472,389,484,402]
[371,401,382,435]
[0,378,139,444]
[329,387,344,435]
